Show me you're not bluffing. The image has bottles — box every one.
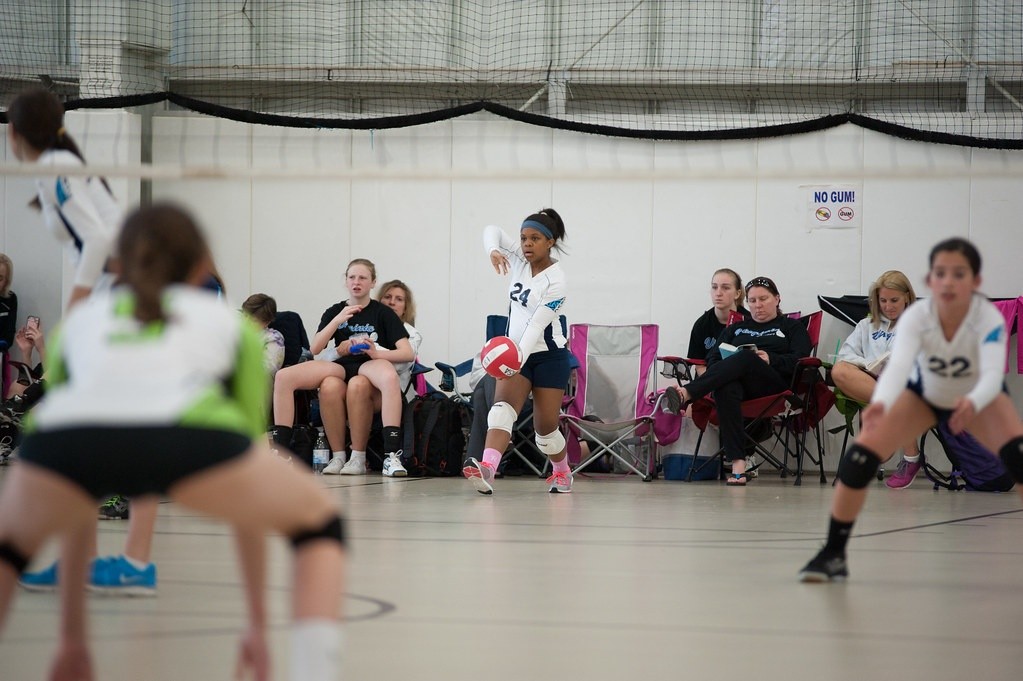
[312,432,329,476]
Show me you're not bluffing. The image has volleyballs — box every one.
[480,336,523,379]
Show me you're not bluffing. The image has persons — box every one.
[269,259,423,479]
[234,292,283,428]
[795,237,1022,584]
[0,198,348,681]
[0,251,53,399]
[831,269,927,492]
[460,205,574,495]
[664,268,814,486]
[0,86,158,598]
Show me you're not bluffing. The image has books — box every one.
[718,342,758,360]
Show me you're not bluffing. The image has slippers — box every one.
[666,386,684,416]
[727,474,751,486]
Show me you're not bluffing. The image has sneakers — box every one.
[746,456,759,478]
[800,546,848,583]
[98,496,128,519]
[885,451,922,489]
[322,457,344,474]
[17,553,161,597]
[271,447,293,466]
[462,456,494,495]
[382,449,408,477]
[340,459,367,474]
[546,470,573,493]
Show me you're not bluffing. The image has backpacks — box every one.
[920,424,1016,493]
[403,392,466,476]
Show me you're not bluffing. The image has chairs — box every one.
[435,315,580,479]
[559,311,870,486]
